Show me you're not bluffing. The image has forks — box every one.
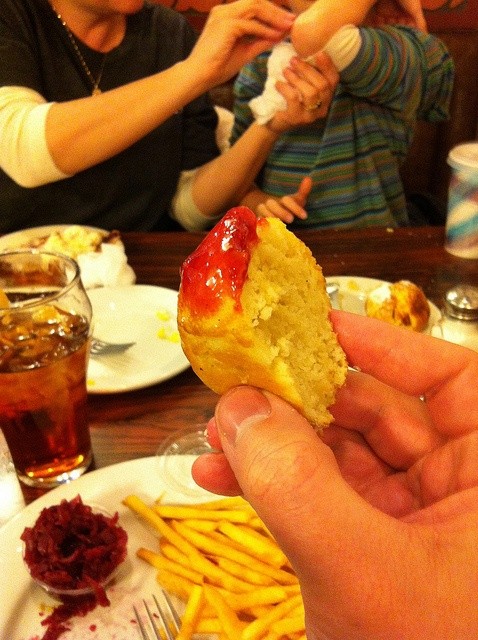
[90,336,134,357]
[133,590,182,636]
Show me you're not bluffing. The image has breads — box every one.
[368,278,432,332]
[35,225,119,260]
[176,207,348,430]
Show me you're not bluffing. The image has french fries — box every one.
[124,494,306,640]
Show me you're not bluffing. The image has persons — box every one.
[191,309,478,640]
[228,0,454,231]
[0,0,339,238]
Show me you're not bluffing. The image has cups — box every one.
[2,254,96,505]
[441,142,478,320]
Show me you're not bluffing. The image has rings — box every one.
[305,100,322,111]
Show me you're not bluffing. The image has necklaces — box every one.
[54,6,121,97]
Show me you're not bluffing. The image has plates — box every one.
[325,275,440,337]
[1,455,240,640]
[87,284,191,393]
